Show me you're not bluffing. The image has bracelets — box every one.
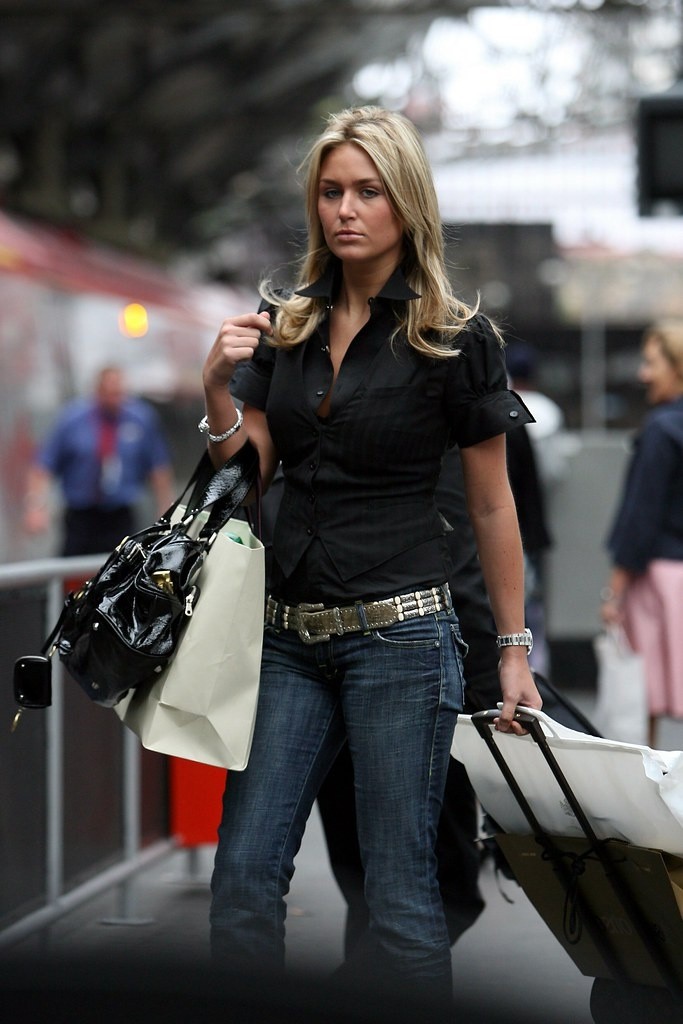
[200,410,242,442]
[497,628,534,657]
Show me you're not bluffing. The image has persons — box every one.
[202,107,544,1024]
[24,363,177,557]
[318,299,683,1024]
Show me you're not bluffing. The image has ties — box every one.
[98,416,118,467]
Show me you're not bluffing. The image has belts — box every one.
[266,581,455,641]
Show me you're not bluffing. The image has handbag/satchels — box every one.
[105,498,267,774]
[15,434,260,714]
[584,623,663,744]
[453,702,683,853]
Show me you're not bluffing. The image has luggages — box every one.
[448,702,683,1023]
